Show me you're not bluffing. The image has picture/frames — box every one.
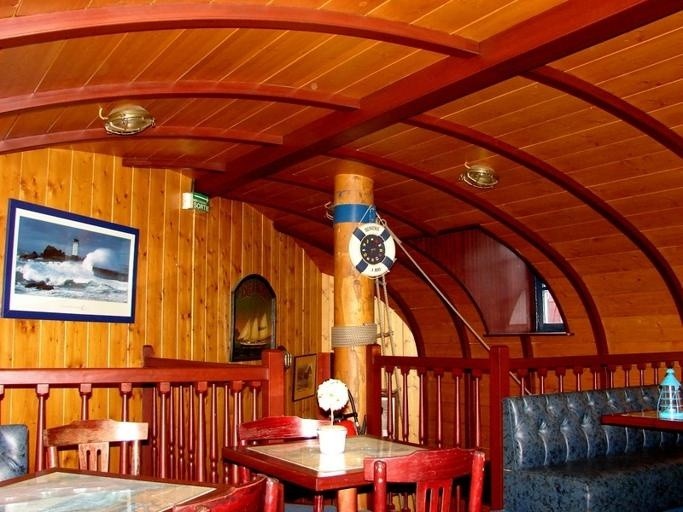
[230,273,276,363]
[0,199,139,323]
[292,353,317,402]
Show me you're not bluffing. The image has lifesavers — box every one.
[349,223,396,277]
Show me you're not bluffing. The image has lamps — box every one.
[99,105,156,136]
[458,162,498,189]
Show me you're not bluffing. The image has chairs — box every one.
[173,477,267,512]
[239,415,359,512]
[43,418,149,476]
[0,424,29,481]
[363,447,490,512]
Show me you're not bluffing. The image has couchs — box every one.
[502,383,683,512]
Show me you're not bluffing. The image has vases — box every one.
[317,426,347,454]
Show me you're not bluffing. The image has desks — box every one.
[601,410,683,434]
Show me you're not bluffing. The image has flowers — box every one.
[318,379,349,426]
